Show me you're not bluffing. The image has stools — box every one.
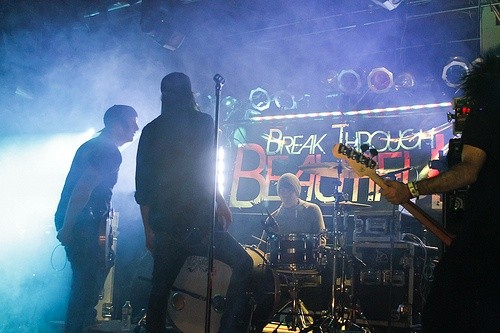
[274,277,323,333]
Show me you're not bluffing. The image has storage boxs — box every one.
[352,212,425,245]
[354,243,424,325]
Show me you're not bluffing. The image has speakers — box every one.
[350,243,436,328]
[442,138,468,220]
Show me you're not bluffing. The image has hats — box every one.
[277,173,301,196]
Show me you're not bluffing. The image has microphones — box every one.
[379,166,412,177]
[264,206,280,232]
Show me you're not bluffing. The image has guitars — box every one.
[99,217,115,289]
[333,143,454,246]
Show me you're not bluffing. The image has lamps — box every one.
[197,54,491,117]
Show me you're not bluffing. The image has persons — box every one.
[378,43,500,333]
[256,170,332,311]
[54,103,140,333]
[133,72,254,333]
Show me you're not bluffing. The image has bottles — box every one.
[121,301,132,331]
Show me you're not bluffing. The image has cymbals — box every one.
[324,200,371,208]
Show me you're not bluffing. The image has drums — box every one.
[167,244,281,333]
[279,253,321,287]
[275,232,322,275]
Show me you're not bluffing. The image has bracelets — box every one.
[407,182,418,197]
